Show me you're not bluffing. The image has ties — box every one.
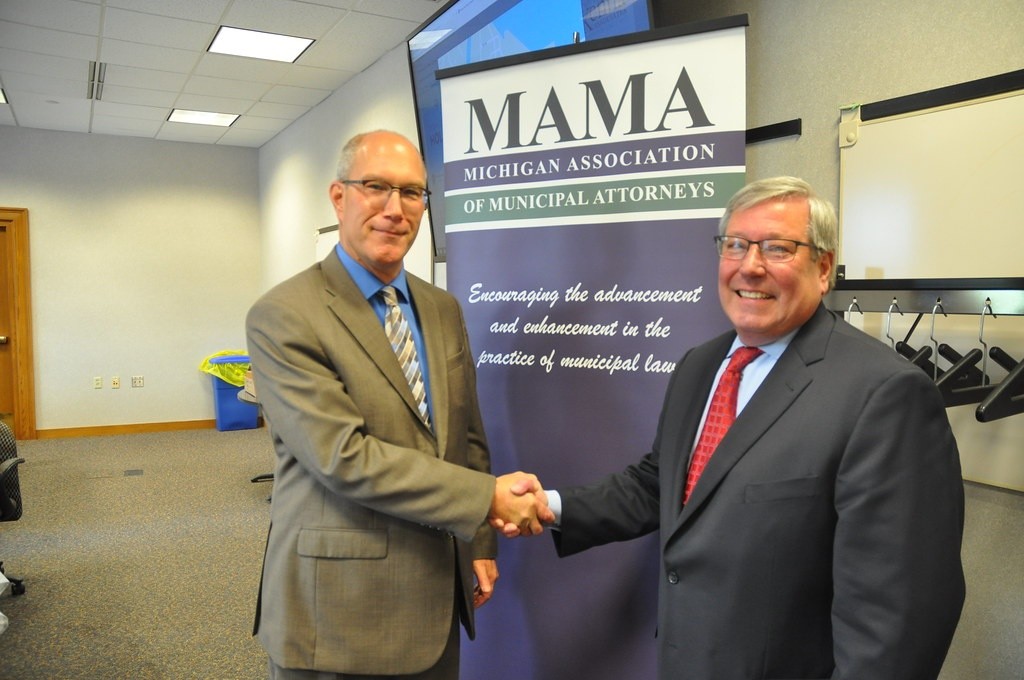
[679,346,764,507]
[377,285,432,432]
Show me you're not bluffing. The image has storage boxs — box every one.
[244,365,256,398]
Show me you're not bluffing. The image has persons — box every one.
[490,176,966,680]
[244,130,556,680]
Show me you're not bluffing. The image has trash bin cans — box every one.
[208,355,258,431]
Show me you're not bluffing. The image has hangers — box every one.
[847,302,1024,423]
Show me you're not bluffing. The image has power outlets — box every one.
[132,376,144,387]
[112,377,120,389]
[94,377,102,389]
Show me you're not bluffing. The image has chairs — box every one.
[0,421,26,596]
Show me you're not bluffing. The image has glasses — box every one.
[713,235,824,263]
[342,179,432,210]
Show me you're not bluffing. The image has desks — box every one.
[238,389,274,503]
